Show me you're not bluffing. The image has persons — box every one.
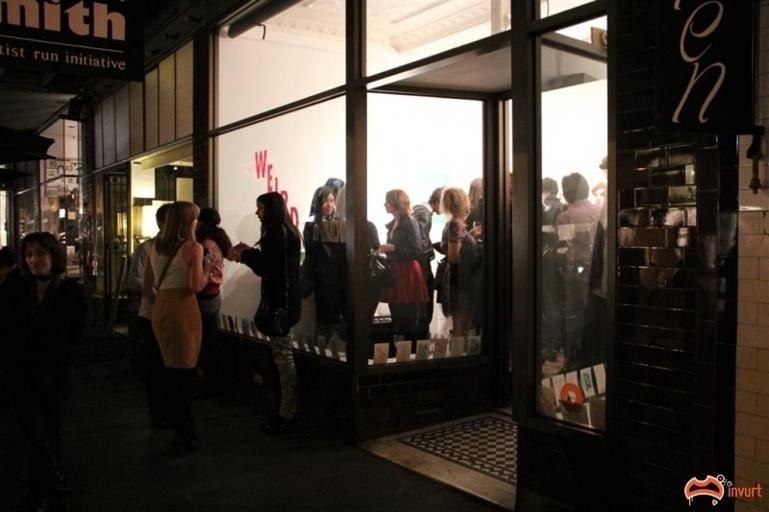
[2,231,90,512]
[122,158,607,451]
[0,244,26,303]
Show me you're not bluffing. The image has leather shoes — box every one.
[170,433,204,452]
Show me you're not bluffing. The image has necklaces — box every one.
[33,272,53,282]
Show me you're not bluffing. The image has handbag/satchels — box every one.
[252,297,292,339]
[368,267,394,293]
[296,254,314,300]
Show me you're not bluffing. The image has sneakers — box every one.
[260,413,299,436]
[28,491,74,512]
